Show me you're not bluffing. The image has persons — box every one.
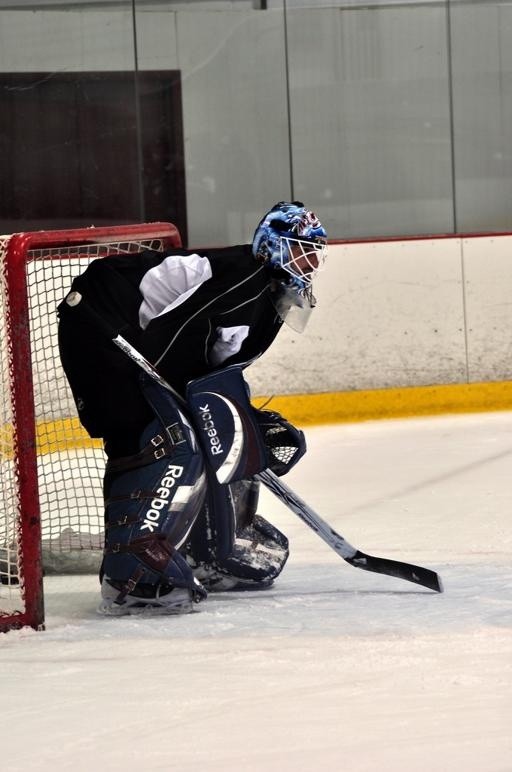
[51,197,331,612]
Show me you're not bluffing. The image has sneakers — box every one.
[188,559,274,592]
[95,573,194,616]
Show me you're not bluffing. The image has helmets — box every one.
[250,200,327,335]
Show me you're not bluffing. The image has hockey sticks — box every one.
[66,291,444,593]
